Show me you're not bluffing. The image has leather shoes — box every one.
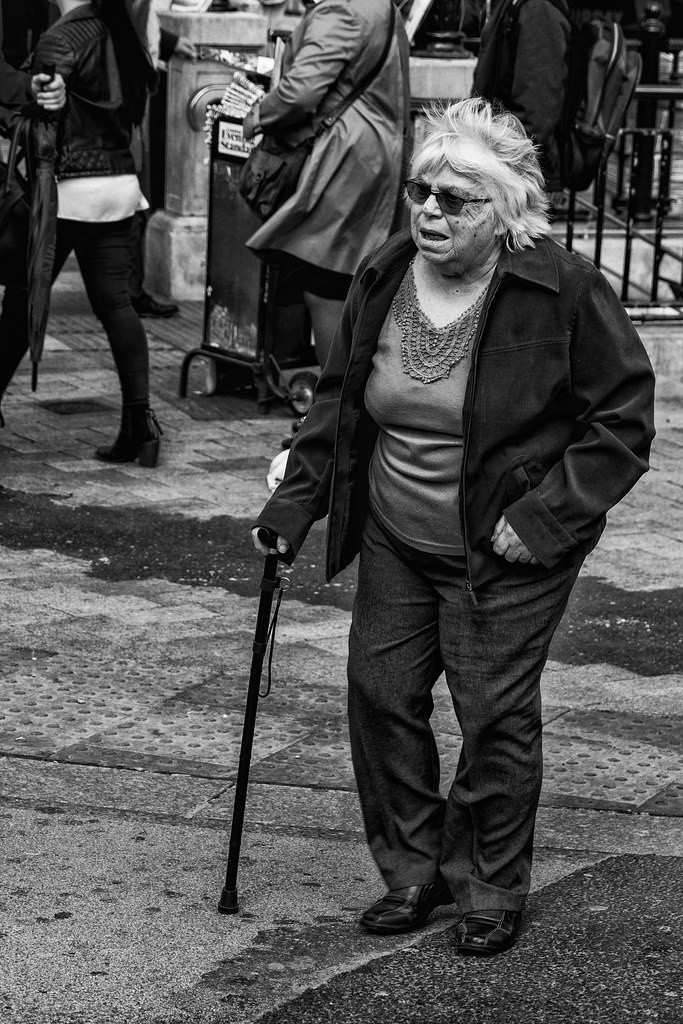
[454,906,521,955]
[359,879,459,934]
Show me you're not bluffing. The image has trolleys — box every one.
[177,103,320,424]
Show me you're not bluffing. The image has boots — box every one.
[96,397,161,466]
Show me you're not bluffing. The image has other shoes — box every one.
[131,290,180,318]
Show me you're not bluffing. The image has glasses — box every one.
[404,175,492,217]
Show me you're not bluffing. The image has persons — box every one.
[471,0,570,191]
[0,0,161,467]
[251,93,656,951]
[243,0,412,445]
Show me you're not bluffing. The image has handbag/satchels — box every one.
[240,127,318,224]
[0,114,36,286]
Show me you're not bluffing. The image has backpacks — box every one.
[505,0,643,193]
